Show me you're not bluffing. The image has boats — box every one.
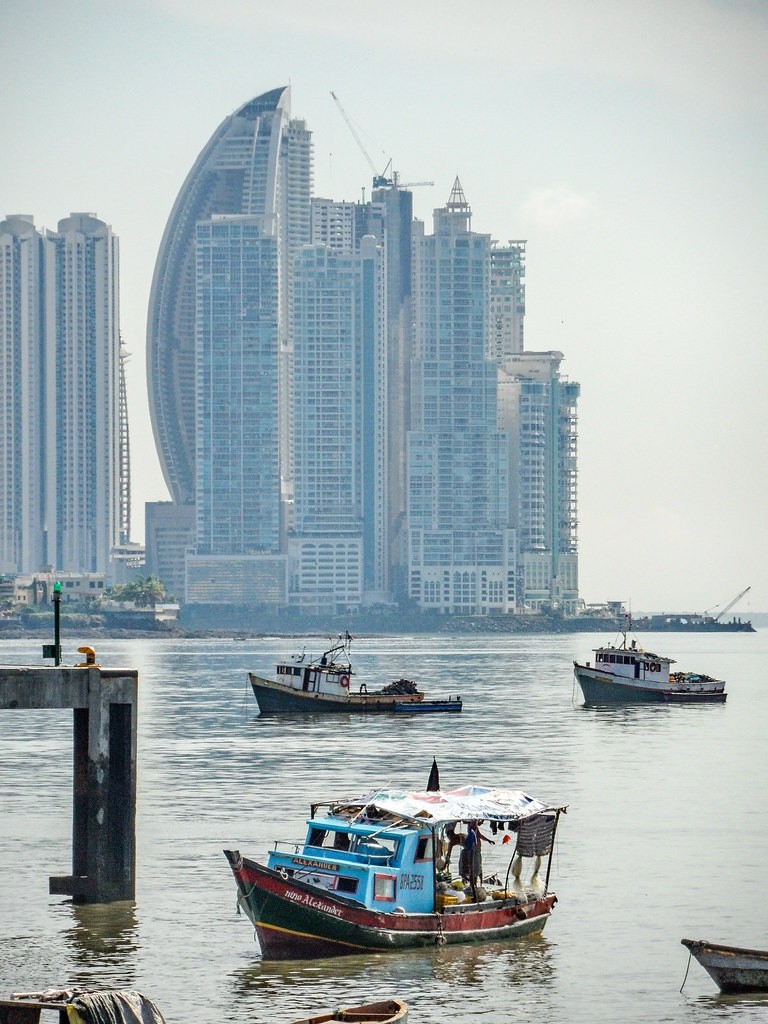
[663,690,728,703]
[290,999,409,1024]
[221,754,570,958]
[573,606,726,703]
[681,938,768,993]
[248,629,464,714]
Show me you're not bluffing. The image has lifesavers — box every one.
[650,662,657,671]
[339,675,349,687]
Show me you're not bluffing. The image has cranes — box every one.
[330,90,436,189]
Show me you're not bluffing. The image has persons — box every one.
[441,820,495,884]
